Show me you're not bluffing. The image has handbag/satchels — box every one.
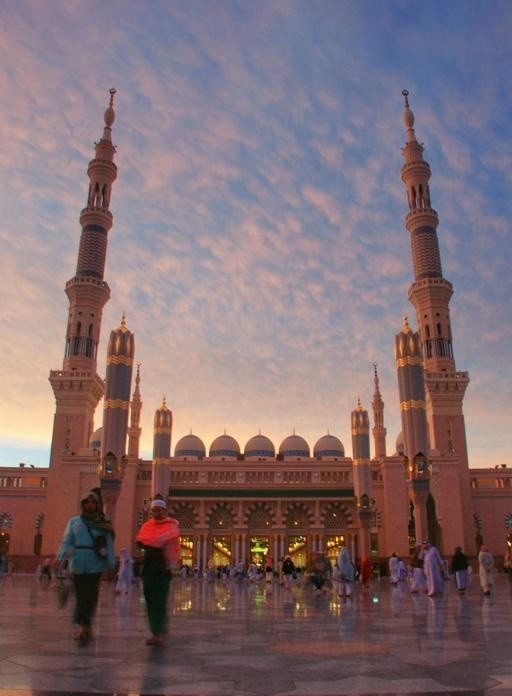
[92,534,107,558]
[137,556,164,580]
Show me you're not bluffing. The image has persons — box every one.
[278,547,371,597]
[452,546,473,593]
[51,484,117,644]
[389,542,449,596]
[193,559,275,583]
[134,493,184,646]
[479,545,495,598]
[115,545,133,595]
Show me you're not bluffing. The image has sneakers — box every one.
[72,627,96,650]
[144,634,159,647]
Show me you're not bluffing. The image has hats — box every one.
[151,499,168,510]
